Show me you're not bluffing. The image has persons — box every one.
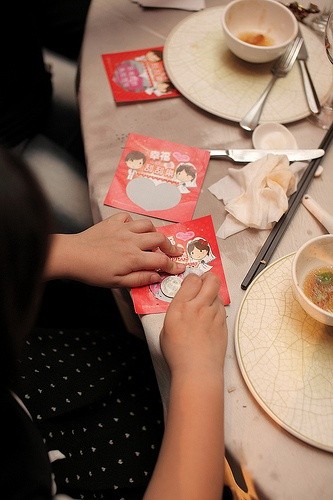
[0,147,229,500]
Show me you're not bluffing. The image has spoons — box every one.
[301,193,333,234]
[251,122,324,178]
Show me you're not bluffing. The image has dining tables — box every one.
[80,0,333,500]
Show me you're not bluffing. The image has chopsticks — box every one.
[240,121,333,291]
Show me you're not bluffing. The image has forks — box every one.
[239,35,305,131]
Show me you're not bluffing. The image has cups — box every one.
[325,9,333,65]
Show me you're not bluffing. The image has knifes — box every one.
[294,25,322,114]
[210,147,326,162]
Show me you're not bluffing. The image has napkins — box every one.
[208,153,308,240]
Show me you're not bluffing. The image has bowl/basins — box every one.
[291,233,333,327]
[220,1,298,64]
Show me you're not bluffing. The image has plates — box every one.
[251,122,298,149]
[162,4,333,125]
[233,249,333,456]
